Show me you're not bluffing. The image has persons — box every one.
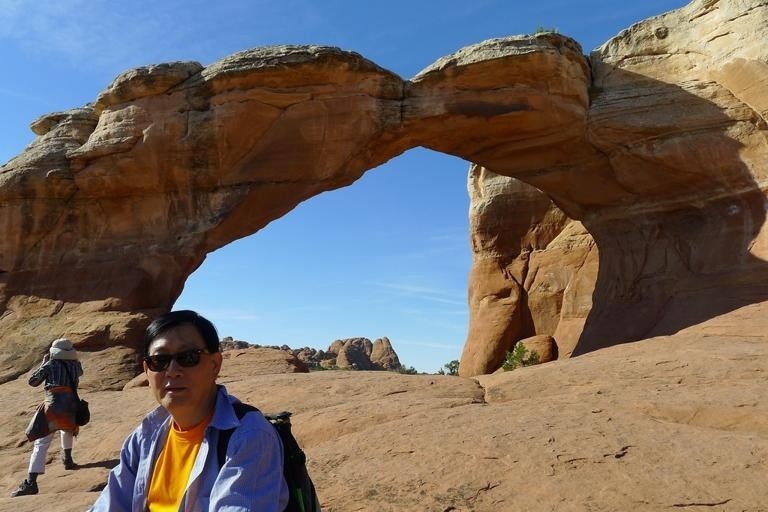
[10,339,83,497]
[85,310,290,512]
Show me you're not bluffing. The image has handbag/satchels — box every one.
[71,399,91,426]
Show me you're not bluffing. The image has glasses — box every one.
[144,348,208,372]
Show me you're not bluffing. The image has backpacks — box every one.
[216,401,322,511]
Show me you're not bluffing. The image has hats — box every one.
[50,338,78,360]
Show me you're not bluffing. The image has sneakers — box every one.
[11,479,38,497]
[63,456,74,470]
